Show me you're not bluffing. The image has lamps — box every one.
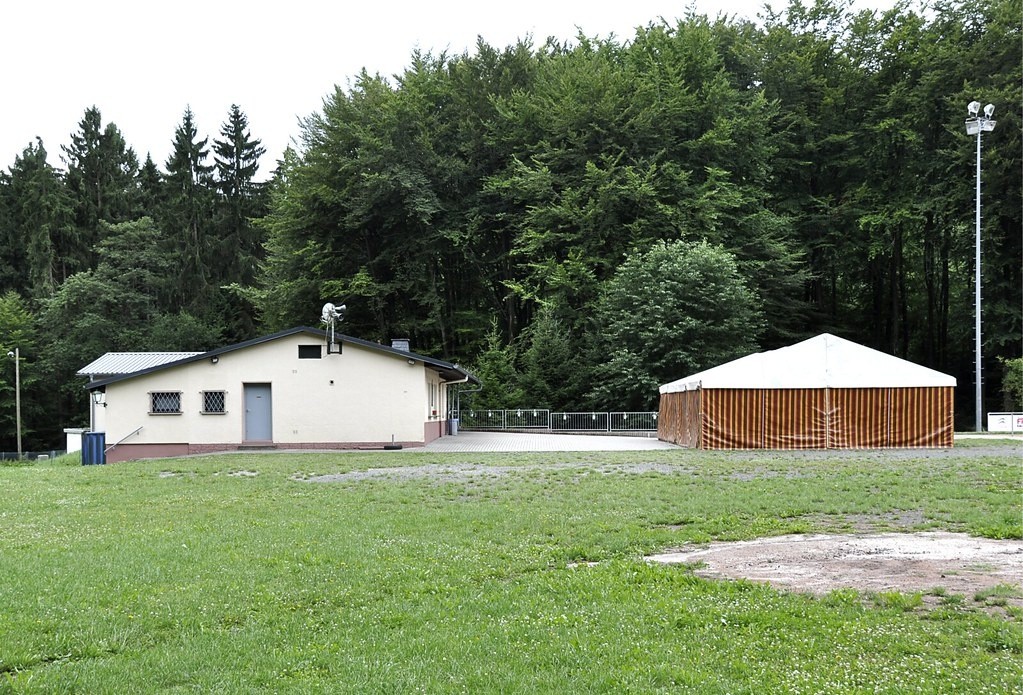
[212,357,218,363]
[92,389,107,407]
[407,359,415,365]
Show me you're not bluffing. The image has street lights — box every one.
[966,99,999,433]
[7,347,23,461]
